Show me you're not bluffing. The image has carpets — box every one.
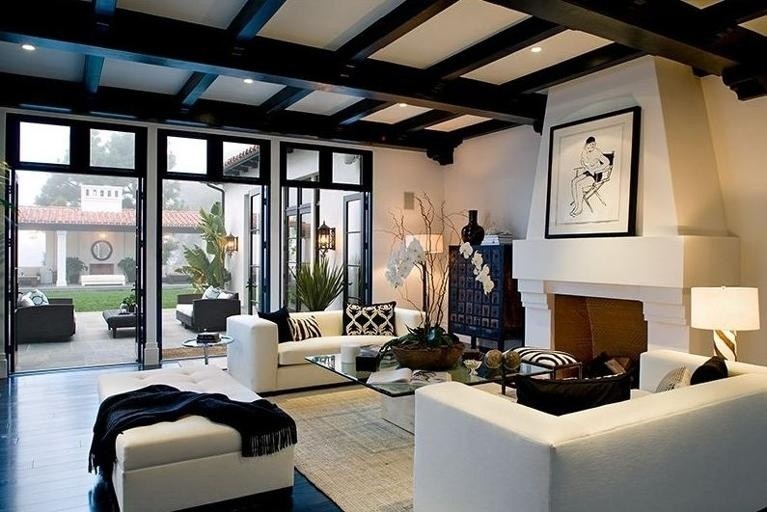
[268,382,519,511]
[161,345,227,359]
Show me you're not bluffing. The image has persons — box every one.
[569,137,610,218]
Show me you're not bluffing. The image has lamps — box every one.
[224,234,239,253]
[401,233,446,313]
[318,222,337,261]
[687,286,762,364]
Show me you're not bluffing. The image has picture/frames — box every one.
[544,106,642,239]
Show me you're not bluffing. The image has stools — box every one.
[502,345,584,393]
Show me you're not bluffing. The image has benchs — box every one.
[96,364,298,511]
[80,274,126,287]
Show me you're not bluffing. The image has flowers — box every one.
[384,187,498,349]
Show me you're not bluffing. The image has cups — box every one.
[339,344,359,364]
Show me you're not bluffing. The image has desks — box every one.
[181,333,233,364]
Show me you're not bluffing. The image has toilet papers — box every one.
[340,342,361,364]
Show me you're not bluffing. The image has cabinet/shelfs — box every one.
[447,245,527,351]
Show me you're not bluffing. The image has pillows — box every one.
[258,308,291,344]
[201,285,234,300]
[515,375,634,416]
[287,315,321,343]
[690,357,729,386]
[17,288,49,307]
[654,366,692,393]
[342,301,398,336]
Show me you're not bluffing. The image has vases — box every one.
[460,209,486,247]
[386,339,465,369]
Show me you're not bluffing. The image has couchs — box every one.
[16,297,77,343]
[224,307,428,395]
[408,349,767,510]
[176,284,241,331]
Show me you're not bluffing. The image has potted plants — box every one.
[123,295,137,312]
[116,257,136,283]
[65,255,87,285]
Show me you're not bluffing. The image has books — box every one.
[367,368,452,388]
